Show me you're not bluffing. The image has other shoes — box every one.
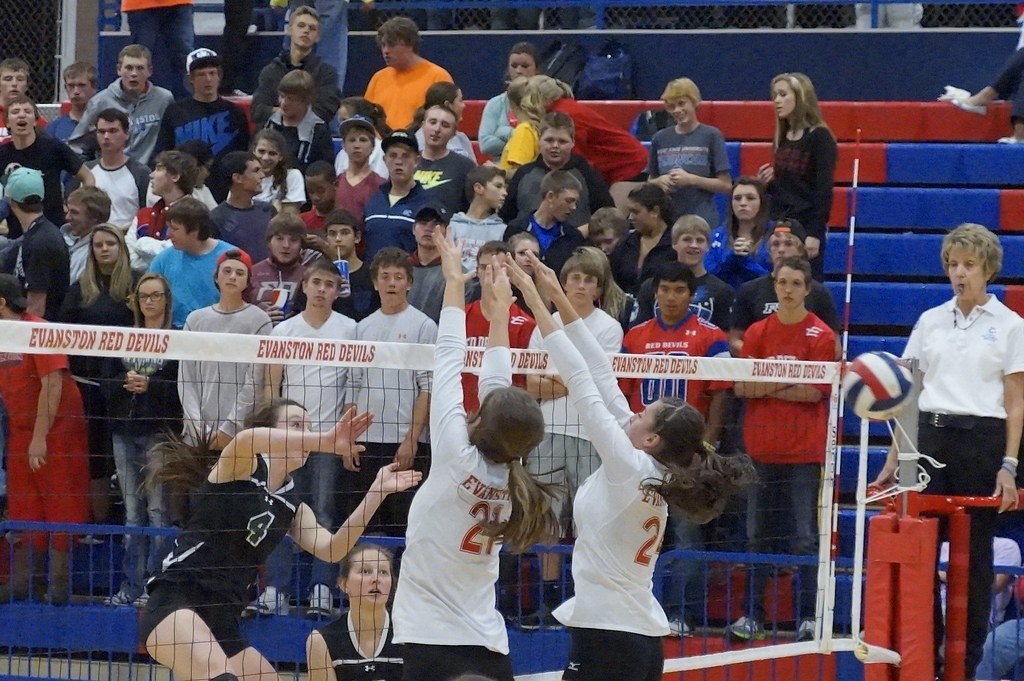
[133,593,149,607]
[307,584,332,619]
[105,590,131,606]
[522,617,564,629]
[246,591,289,615]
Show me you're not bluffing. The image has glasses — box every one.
[137,290,165,302]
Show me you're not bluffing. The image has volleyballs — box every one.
[843,351,915,421]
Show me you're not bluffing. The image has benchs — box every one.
[456,97,1024,631]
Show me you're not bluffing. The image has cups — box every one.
[271,289,290,319]
[331,259,351,298]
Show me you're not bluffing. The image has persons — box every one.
[756,73,839,285]
[390,224,545,679]
[1,1,1024,680]
[0,274,91,606]
[358,246,439,575]
[644,77,733,230]
[138,397,424,681]
[102,274,183,606]
[244,259,358,621]
[871,223,1024,681]
[178,249,274,455]
[494,244,754,681]
[158,47,249,207]
[307,541,410,680]
[362,17,456,132]
[68,44,175,174]
[249,5,341,129]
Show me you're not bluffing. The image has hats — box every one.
[5,167,45,203]
[340,113,375,129]
[381,131,418,154]
[414,202,450,223]
[186,47,217,74]
[770,218,807,244]
[217,249,252,268]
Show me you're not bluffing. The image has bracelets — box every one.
[1001,462,1017,477]
[1002,456,1018,466]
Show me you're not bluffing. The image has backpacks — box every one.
[573,47,643,100]
[539,41,583,85]
[637,110,674,141]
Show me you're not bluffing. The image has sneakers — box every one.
[798,621,816,641]
[731,616,769,641]
[668,618,693,638]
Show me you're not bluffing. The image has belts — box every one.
[919,411,1004,431]
